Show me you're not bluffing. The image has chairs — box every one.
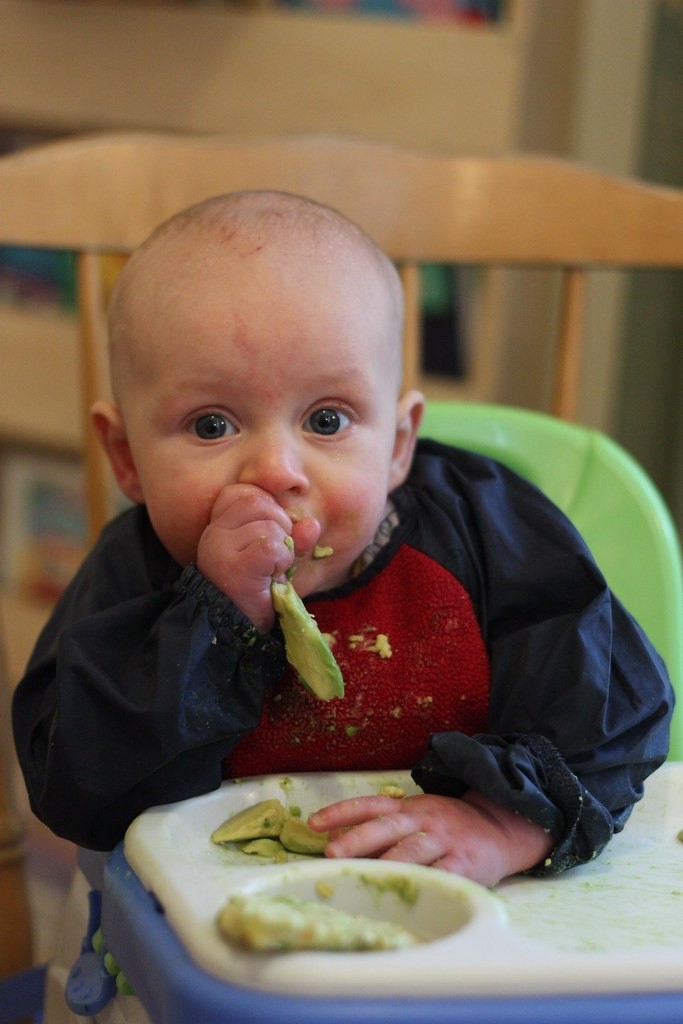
[414,398,683,765]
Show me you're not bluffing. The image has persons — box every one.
[10,190,677,886]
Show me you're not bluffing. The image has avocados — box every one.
[214,585,407,855]
